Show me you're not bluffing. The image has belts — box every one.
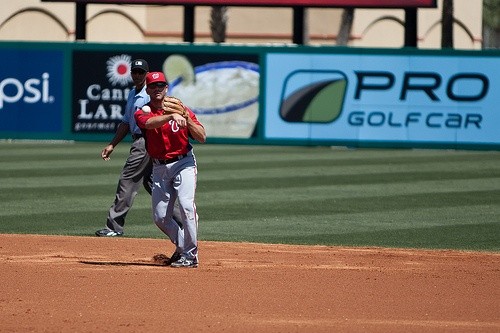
[153,153,187,164]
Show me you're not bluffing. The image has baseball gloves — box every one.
[161,95,187,121]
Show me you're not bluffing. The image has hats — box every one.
[145,71,167,87]
[131,59,150,72]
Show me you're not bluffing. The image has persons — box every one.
[96,59,200,237]
[134,72,206,268]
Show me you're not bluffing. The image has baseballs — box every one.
[142,105,152,115]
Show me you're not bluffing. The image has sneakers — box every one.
[95,225,124,237]
[170,251,199,268]
